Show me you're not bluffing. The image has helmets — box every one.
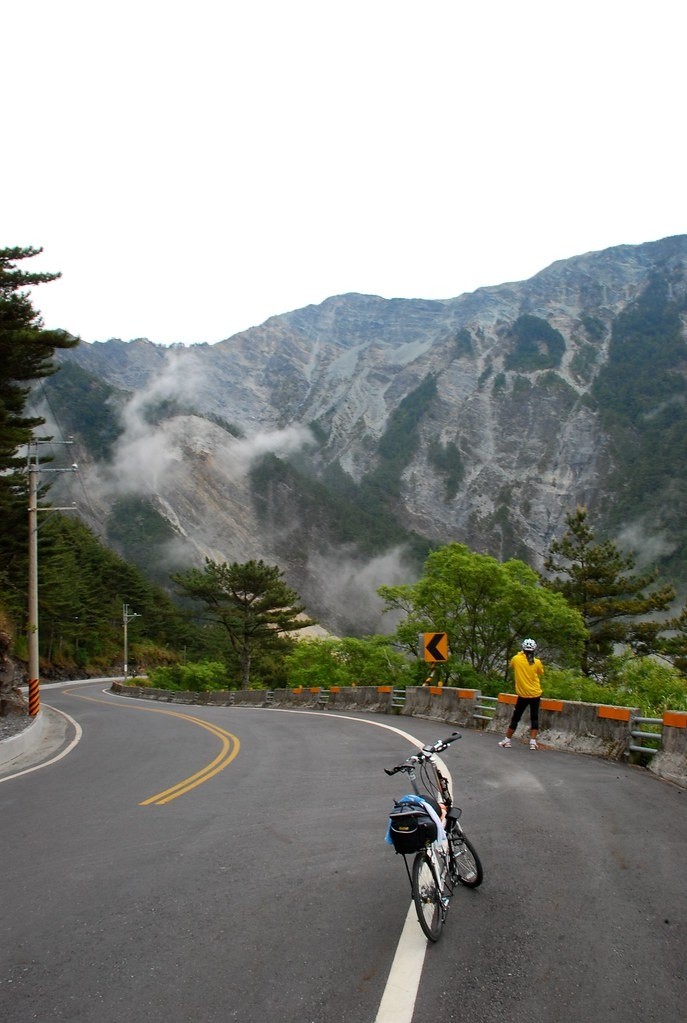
[522,639,537,652]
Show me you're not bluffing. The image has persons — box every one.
[498,639,545,750]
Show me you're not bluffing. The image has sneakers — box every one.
[530,744,538,750]
[499,740,512,747]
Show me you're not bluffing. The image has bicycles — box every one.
[385,729,485,943]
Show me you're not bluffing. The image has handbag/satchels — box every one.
[388,804,438,855]
